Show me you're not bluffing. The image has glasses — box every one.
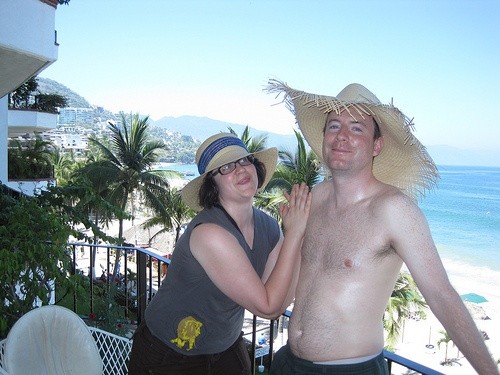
[213,155,254,177]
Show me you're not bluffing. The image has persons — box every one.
[128,132,312,375]
[269,84,500,375]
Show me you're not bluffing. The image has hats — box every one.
[181,132,278,212]
[262,75,442,205]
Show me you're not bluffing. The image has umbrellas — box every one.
[461,293,488,310]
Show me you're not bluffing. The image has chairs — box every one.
[4,305,103,375]
